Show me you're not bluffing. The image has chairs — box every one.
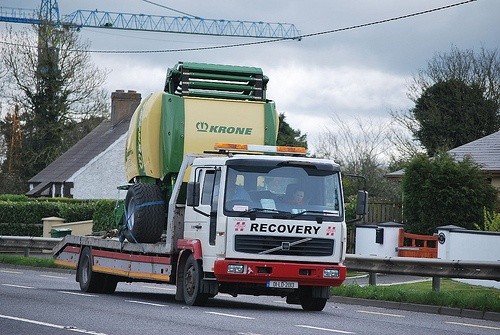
[218,183,309,215]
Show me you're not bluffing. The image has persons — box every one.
[288,188,310,205]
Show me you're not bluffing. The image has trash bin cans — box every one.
[50,226,73,240]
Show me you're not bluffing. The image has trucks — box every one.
[50,141,370,313]
